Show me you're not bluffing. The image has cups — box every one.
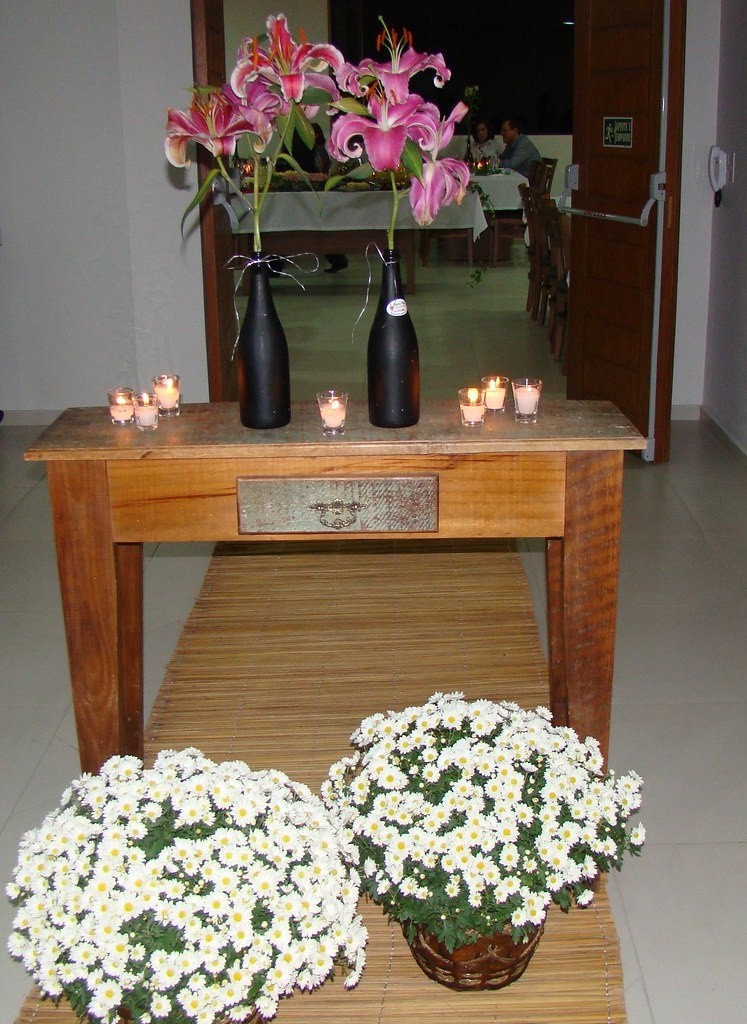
[457,387,487,428]
[151,374,182,417]
[107,387,135,426]
[481,376,510,414]
[131,393,159,431]
[315,389,350,437]
[512,378,542,423]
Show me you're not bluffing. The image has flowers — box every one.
[4,748,370,1024]
[325,16,495,287]
[321,691,645,957]
[164,13,343,250]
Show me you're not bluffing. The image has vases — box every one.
[401,903,548,992]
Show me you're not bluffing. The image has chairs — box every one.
[492,157,558,268]
[518,184,571,376]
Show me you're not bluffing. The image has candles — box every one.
[460,387,484,421]
[135,392,158,426]
[516,378,540,414]
[155,378,179,408]
[320,400,345,427]
[485,376,506,407]
[110,396,134,420]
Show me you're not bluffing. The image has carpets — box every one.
[15,540,626,1024]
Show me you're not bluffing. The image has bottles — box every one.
[463,143,473,166]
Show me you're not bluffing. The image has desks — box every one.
[470,168,530,262]
[24,401,647,779]
[231,189,489,295]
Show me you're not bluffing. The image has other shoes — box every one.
[324,263,346,273]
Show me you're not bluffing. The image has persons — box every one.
[256,123,348,277]
[469,122,505,159]
[478,119,545,261]
